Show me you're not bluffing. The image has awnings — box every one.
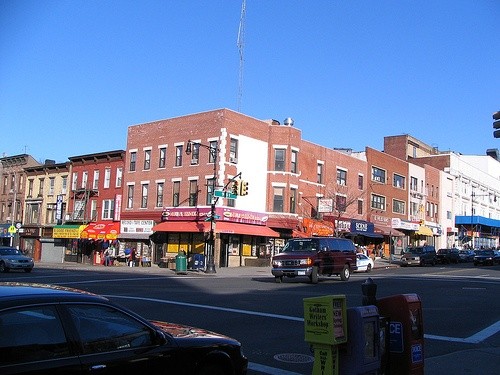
[355,231,385,238]
[151,221,281,238]
[373,226,405,237]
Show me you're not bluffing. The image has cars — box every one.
[0,281,249,375]
[436,249,457,264]
[458,250,475,262]
[354,253,374,273]
[474,250,500,266]
[401,246,436,267]
[0,247,34,272]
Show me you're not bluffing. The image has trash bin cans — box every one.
[340,305,383,375]
[175,255,188,275]
[375,293,424,375]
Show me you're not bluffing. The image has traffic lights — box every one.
[193,144,199,156]
[493,111,500,138]
[186,143,191,155]
[232,180,240,196]
[241,180,249,196]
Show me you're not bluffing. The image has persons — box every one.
[129,247,137,267]
[103,244,117,266]
[179,246,186,256]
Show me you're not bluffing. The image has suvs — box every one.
[271,238,358,283]
